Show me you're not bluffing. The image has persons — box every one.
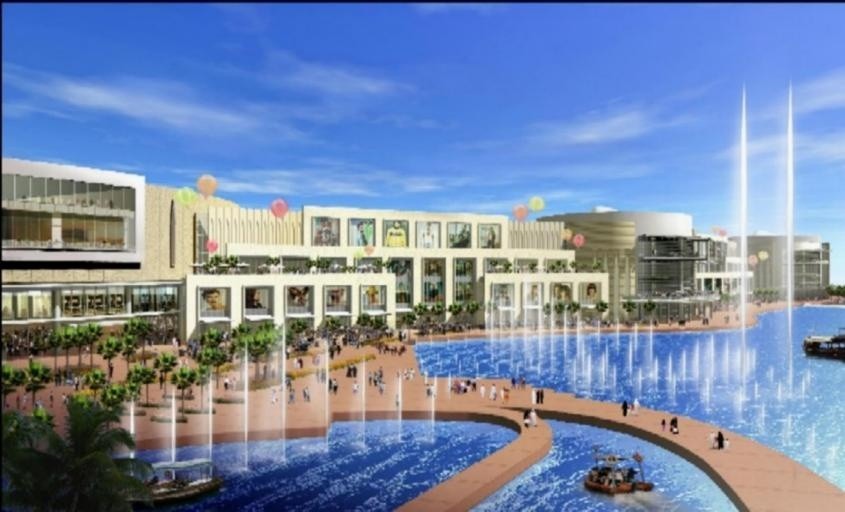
[708,429,718,449]
[527,285,541,306]
[420,220,441,250]
[200,288,225,312]
[585,283,599,303]
[494,285,512,306]
[661,417,667,433]
[486,224,499,248]
[385,220,407,249]
[627,403,634,415]
[716,431,725,450]
[673,423,677,435]
[351,216,375,247]
[550,284,562,301]
[559,284,572,304]
[423,375,545,428]
[16,365,81,409]
[723,438,730,449]
[670,415,679,432]
[221,321,416,409]
[109,361,114,378]
[244,287,267,310]
[633,397,641,415]
[452,222,471,248]
[590,452,640,489]
[314,215,335,246]
[622,399,629,416]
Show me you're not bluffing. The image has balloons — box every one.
[749,254,758,267]
[513,201,529,225]
[573,232,585,248]
[528,195,545,211]
[195,174,217,200]
[711,224,720,235]
[174,185,197,214]
[271,196,289,221]
[206,239,218,258]
[759,250,769,261]
[718,228,727,238]
